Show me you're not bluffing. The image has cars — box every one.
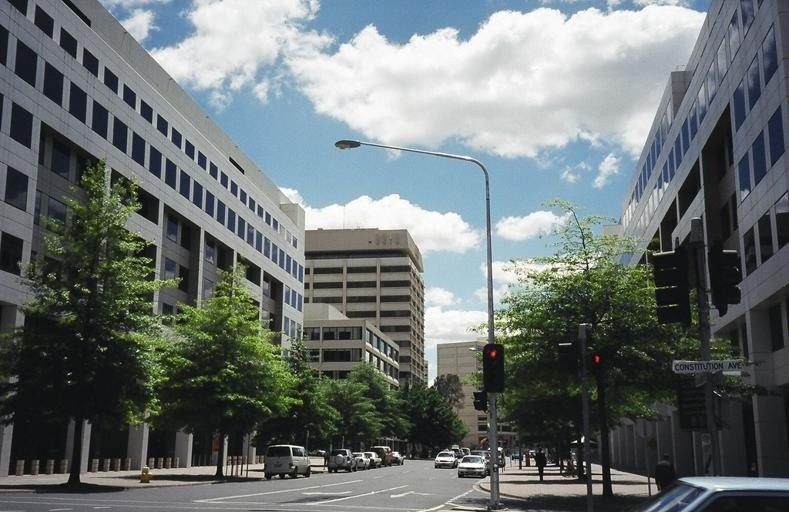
[432,443,536,477]
[309,445,405,473]
[622,475,789,512]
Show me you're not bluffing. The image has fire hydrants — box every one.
[138,464,152,485]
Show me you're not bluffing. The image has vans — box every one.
[262,443,313,479]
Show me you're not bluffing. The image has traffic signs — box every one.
[670,357,746,376]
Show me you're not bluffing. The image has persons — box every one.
[655,453,676,492]
[523,446,531,466]
[533,447,547,483]
[322,450,330,470]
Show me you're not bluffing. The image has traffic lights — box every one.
[708,237,743,319]
[651,236,693,332]
[481,343,506,394]
[472,389,487,411]
[586,348,608,375]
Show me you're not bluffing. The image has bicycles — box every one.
[560,460,588,480]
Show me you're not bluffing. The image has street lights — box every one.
[332,139,515,511]
